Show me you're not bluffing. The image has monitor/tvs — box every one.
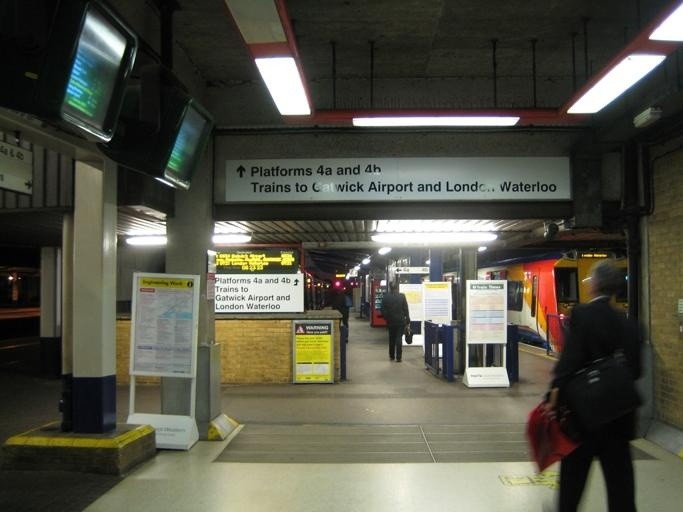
[0,0,140,145]
[96,84,215,192]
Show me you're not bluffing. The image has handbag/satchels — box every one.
[560,350,642,433]
[405,323,413,344]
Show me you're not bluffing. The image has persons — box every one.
[381,281,410,362]
[549,259,647,512]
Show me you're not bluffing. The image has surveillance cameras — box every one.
[543,219,559,241]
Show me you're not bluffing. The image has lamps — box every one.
[562,42,671,118]
[250,46,313,117]
[316,107,564,128]
[631,0,682,56]
[225,1,295,51]
